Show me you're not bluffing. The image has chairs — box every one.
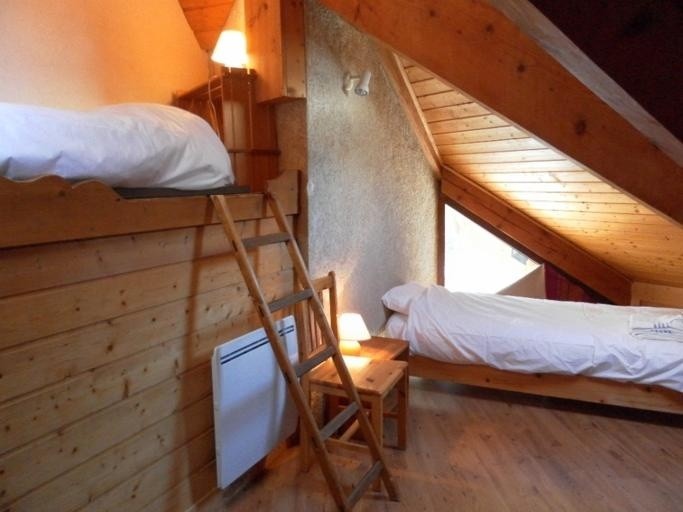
[294,271,409,491]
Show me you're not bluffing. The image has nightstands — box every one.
[328,337,410,413]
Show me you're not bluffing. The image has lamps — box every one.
[211,29,248,74]
[336,313,371,353]
[343,70,372,96]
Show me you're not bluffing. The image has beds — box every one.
[381,281,683,419]
[1,95,300,251]
